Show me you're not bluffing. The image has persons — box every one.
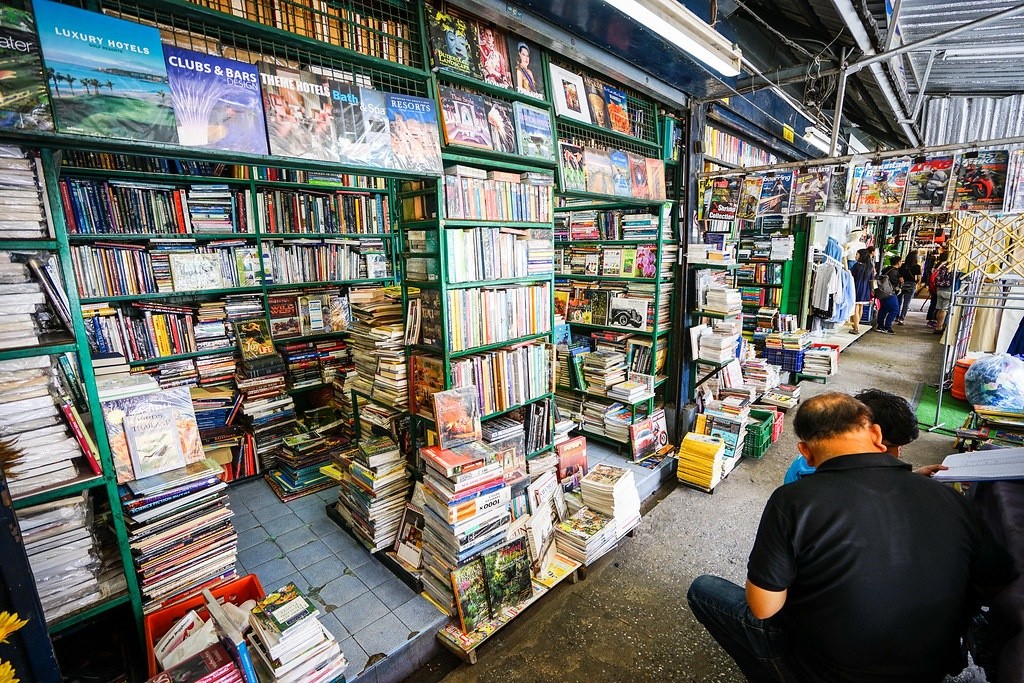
[515,41,545,100]
[844,227,866,271]
[686,386,985,683]
[924,248,952,335]
[477,26,508,90]
[852,246,921,338]
[438,19,481,80]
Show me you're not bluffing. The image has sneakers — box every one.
[882,326,896,335]
[875,325,884,332]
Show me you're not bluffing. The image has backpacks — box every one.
[934,263,953,289]
[874,268,895,299]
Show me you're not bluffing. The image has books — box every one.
[0,0,681,683]
[933,405,1024,483]
[677,215,838,490]
[697,124,1024,222]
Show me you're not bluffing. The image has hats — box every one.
[518,42,530,55]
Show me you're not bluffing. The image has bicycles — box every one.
[875,170,899,204]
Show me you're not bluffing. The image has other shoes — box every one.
[933,326,945,335]
[849,325,860,335]
[898,320,905,325]
[927,320,938,328]
[895,318,899,322]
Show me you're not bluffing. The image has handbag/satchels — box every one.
[870,280,878,289]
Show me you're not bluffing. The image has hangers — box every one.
[825,256,844,270]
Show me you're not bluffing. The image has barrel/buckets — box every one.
[951,359,972,401]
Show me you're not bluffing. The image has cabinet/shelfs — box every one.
[910,214,952,251]
[0,149,1024,683]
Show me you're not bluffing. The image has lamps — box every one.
[606,0,743,76]
[943,46,1024,60]
[802,125,842,159]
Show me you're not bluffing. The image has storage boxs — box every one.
[743,408,773,461]
[810,343,840,367]
[762,345,809,373]
[771,412,785,443]
[144,573,266,677]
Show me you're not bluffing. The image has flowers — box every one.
[0,611,29,683]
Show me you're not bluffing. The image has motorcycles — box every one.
[917,170,945,204]
